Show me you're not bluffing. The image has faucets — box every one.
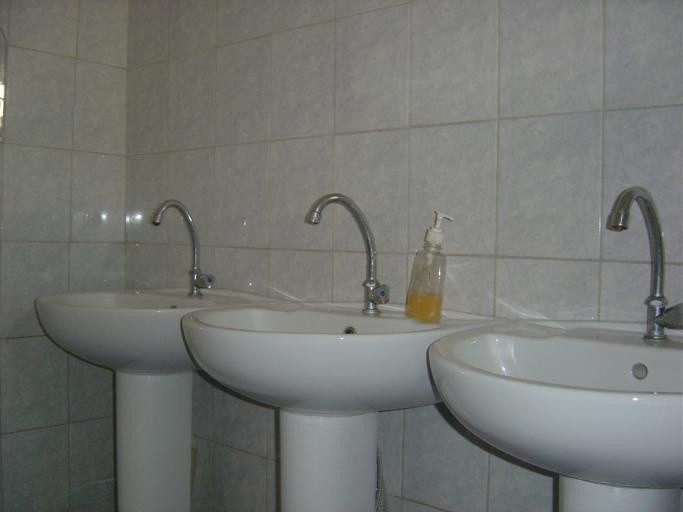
[149,197,214,297]
[604,185,668,344]
[303,191,389,317]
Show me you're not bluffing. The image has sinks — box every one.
[426,319,682,492]
[33,290,280,377]
[179,306,496,414]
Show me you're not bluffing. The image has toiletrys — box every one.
[407,209,454,324]
